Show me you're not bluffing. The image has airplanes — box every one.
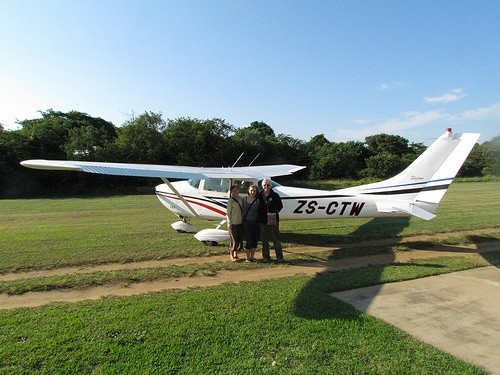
[19,128,481,246]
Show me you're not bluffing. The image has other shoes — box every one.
[231,258,237,262]
[235,256,242,261]
[273,258,283,264]
[245,258,250,262]
[261,258,272,262]
[251,257,256,262]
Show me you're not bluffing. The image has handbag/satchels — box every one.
[267,213,277,225]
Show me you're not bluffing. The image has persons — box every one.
[259,177,284,264]
[226,184,243,262]
[243,185,261,263]
